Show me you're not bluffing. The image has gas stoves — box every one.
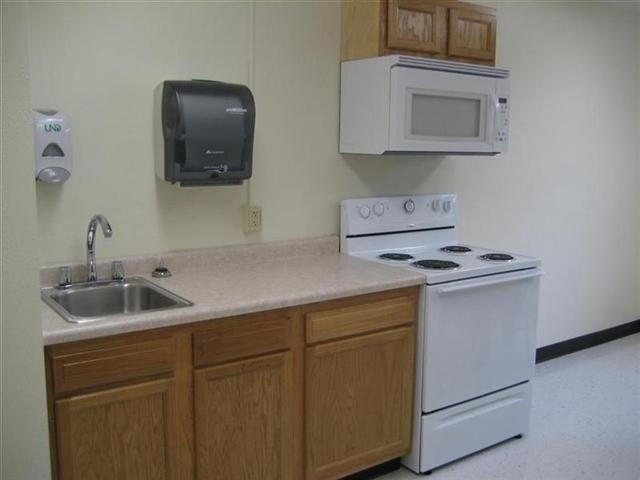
[349,239,540,286]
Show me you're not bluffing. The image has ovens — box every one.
[417,266,541,474]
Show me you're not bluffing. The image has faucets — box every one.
[85,212,114,281]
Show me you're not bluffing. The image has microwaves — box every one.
[336,54,513,158]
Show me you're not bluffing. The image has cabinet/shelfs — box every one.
[341,0,496,68]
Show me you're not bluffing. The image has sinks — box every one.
[45,279,184,318]
